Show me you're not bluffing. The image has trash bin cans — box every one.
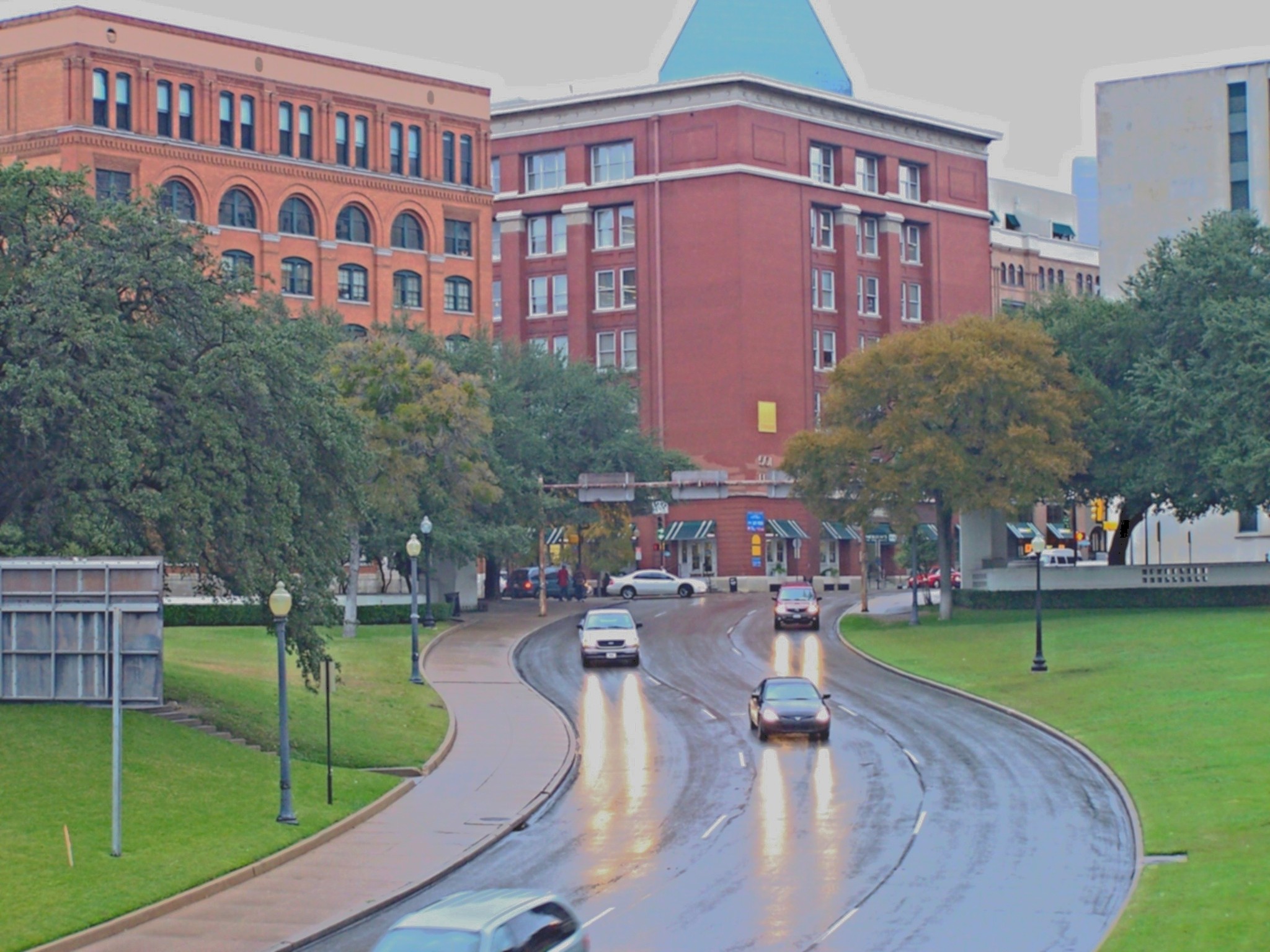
[729,577,738,591]
[444,592,461,616]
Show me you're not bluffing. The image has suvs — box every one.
[577,609,643,667]
[370,889,589,952]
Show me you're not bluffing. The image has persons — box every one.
[573,565,588,603]
[602,573,610,597]
[557,565,570,601]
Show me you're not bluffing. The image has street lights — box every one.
[269,580,299,825]
[406,533,426,684]
[421,515,435,627]
[1031,533,1048,671]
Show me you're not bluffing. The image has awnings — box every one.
[867,521,897,545]
[768,519,809,539]
[1006,520,1042,541]
[912,521,940,541]
[1048,522,1075,539]
[824,521,862,541]
[660,519,717,541]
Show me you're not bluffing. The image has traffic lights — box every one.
[658,528,665,541]
[1076,532,1085,542]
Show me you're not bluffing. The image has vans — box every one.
[507,567,576,599]
[1027,549,1082,567]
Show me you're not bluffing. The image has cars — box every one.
[606,569,708,600]
[772,582,823,631]
[748,676,832,742]
[908,565,961,589]
[480,571,509,596]
